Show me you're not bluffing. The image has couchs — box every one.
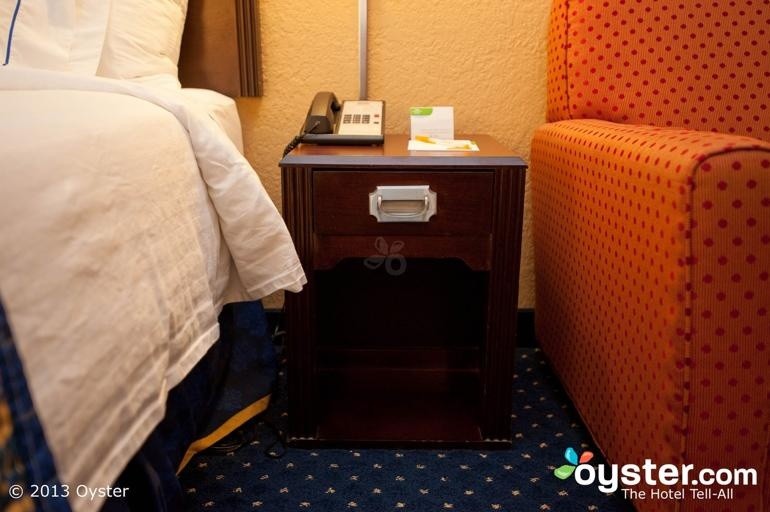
[530,0,770,512]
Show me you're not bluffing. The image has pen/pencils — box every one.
[416,136,448,146]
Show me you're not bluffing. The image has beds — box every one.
[0,0,264,511]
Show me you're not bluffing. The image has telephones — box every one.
[283,91,385,158]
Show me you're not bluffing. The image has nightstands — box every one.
[263,133,529,450]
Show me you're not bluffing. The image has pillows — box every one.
[0,0,110,79]
[97,1,188,81]
